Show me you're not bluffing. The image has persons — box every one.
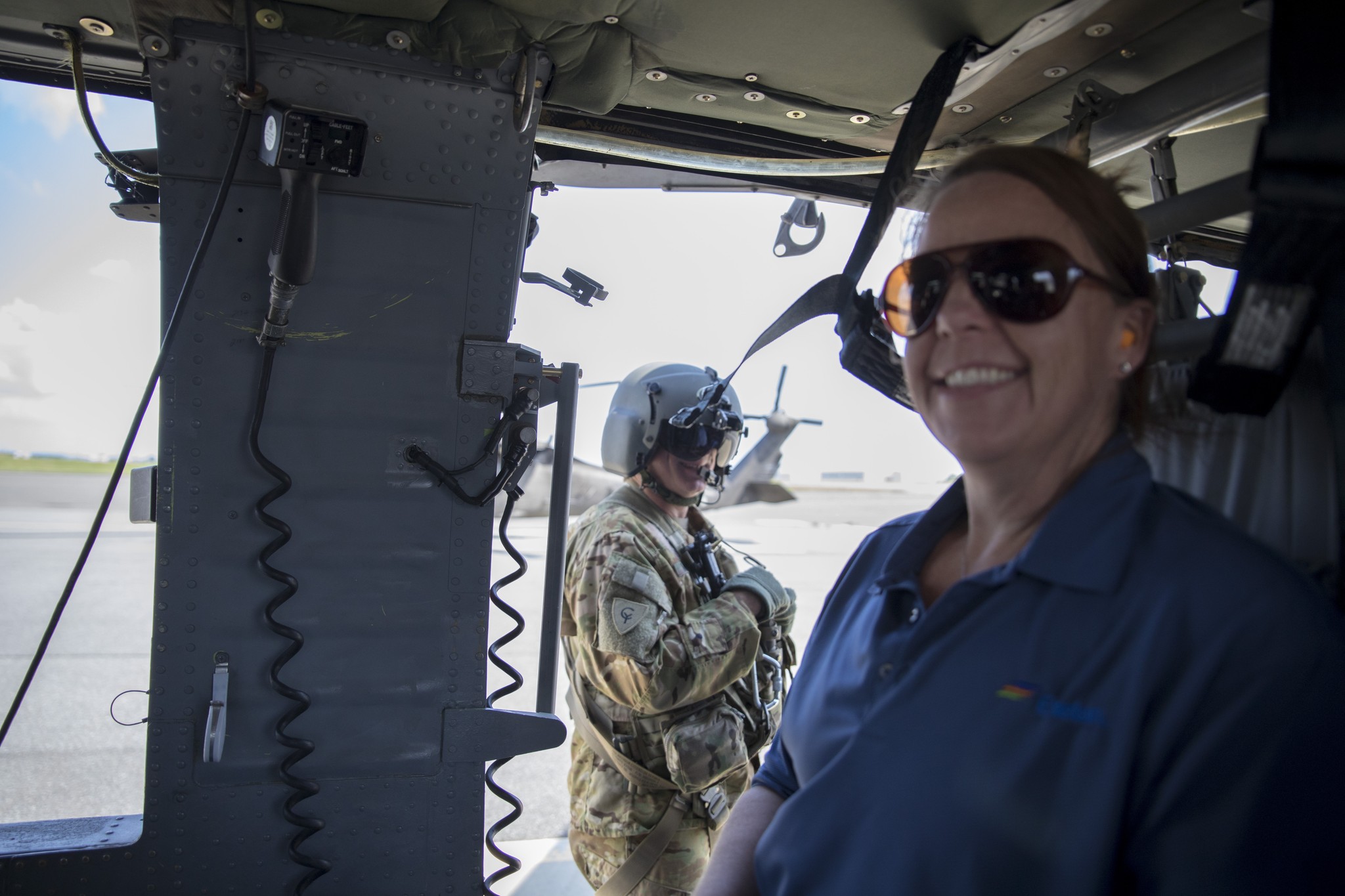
[560,361,798,895]
[690,147,1345,896]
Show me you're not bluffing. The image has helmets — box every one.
[601,361,743,478]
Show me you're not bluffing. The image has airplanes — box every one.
[495,366,823,522]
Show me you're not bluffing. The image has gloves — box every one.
[772,587,798,637]
[720,566,790,618]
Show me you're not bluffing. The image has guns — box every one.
[679,525,784,699]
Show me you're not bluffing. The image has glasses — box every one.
[878,234,1127,340]
[656,421,738,468]
